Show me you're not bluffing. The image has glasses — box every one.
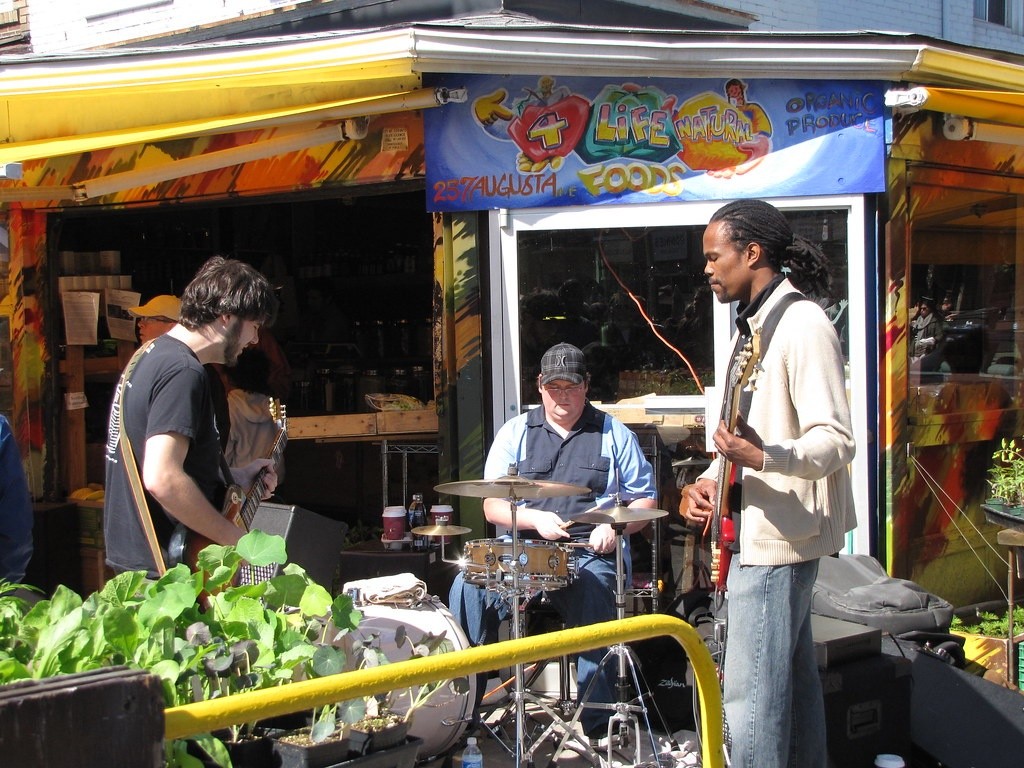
[545,380,585,391]
[140,316,165,322]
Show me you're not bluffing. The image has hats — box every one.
[541,343,587,385]
[128,294,182,321]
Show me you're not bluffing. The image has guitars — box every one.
[169,395,289,618]
[709,331,764,593]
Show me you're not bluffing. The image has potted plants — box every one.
[47,527,473,767]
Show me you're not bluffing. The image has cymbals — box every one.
[568,507,670,525]
[432,476,593,500]
[410,525,473,536]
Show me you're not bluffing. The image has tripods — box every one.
[462,490,682,768]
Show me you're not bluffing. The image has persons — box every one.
[910,299,960,358]
[517,257,715,403]
[448,343,658,753]
[100,255,293,582]
[0,414,33,584]
[678,198,857,768]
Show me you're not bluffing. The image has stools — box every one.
[490,608,579,740]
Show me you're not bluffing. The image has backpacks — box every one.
[810,552,957,638]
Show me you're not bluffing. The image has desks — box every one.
[996,526,1024,684]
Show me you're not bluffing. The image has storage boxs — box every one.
[78,502,112,589]
[283,407,438,439]
[810,612,882,671]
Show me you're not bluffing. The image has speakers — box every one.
[238,502,348,595]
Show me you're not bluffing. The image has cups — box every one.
[429,505,454,547]
[383,505,407,540]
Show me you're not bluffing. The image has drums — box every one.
[285,591,478,765]
[462,538,574,591]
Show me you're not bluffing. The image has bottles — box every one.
[409,494,428,552]
[618,368,713,399]
[137,219,212,248]
[385,243,424,275]
[351,318,433,359]
[300,367,434,413]
[298,252,335,282]
